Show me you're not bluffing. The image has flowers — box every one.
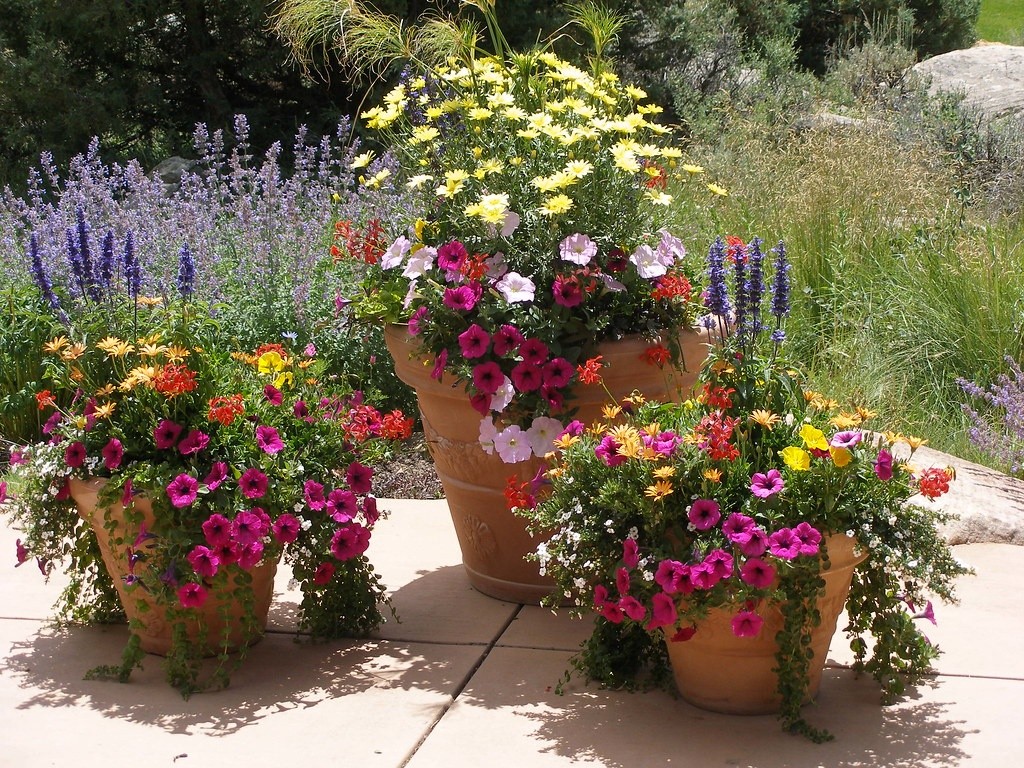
[0,327,413,698]
[331,54,978,744]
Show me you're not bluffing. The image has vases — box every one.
[384,309,736,608]
[653,519,869,715]
[67,472,286,661]
